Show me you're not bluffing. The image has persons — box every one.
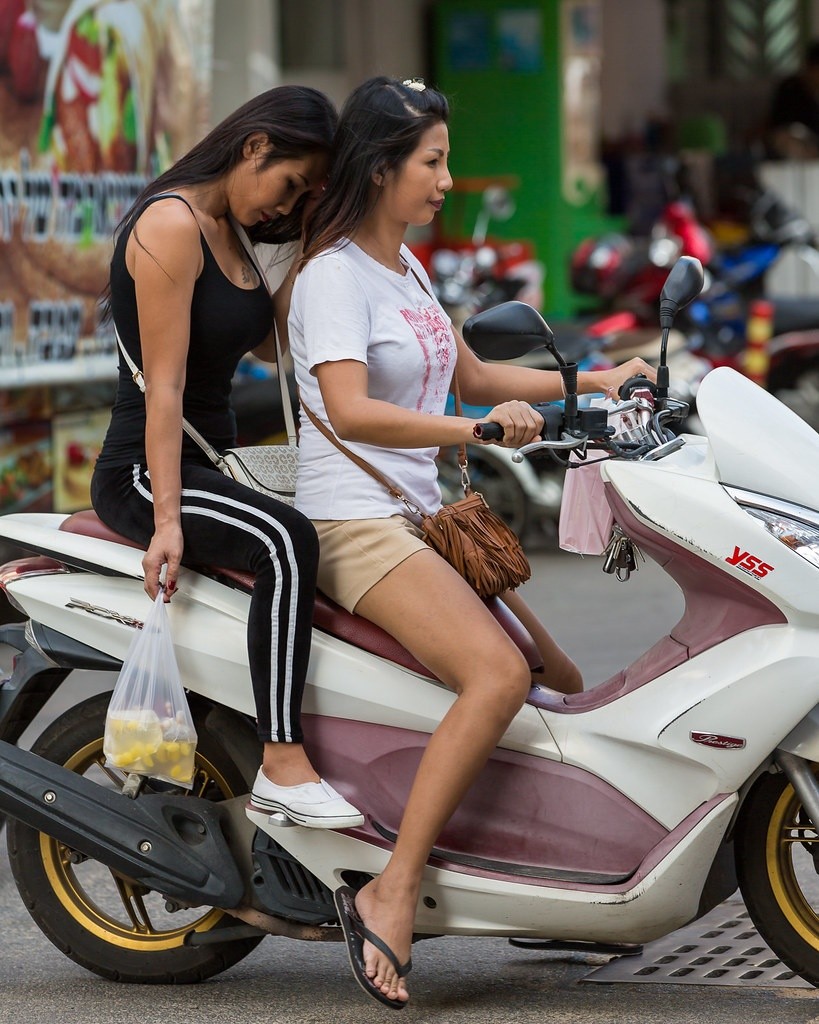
[628,114,795,243]
[90,83,365,830]
[284,76,657,1010]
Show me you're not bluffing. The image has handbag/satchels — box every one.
[103,580,198,790]
[557,394,635,555]
[224,445,299,507]
[419,490,532,596]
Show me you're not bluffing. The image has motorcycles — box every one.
[0,254,819,989]
[417,177,819,554]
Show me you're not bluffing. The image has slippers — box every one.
[333,884,414,1011]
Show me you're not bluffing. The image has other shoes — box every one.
[248,766,370,830]
